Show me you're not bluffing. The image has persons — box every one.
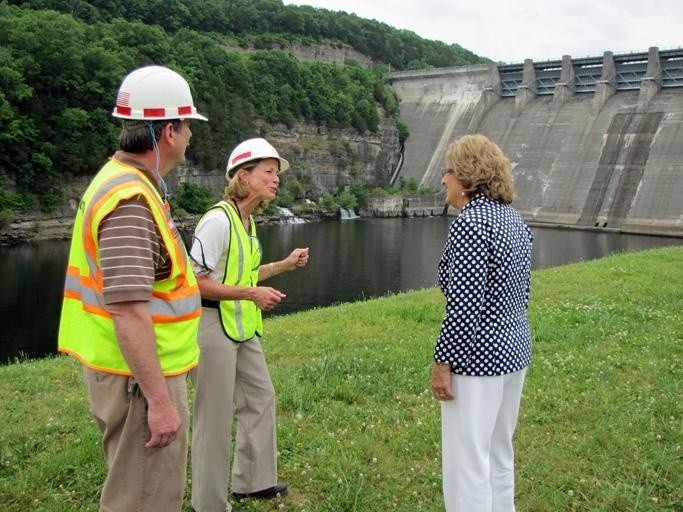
[190,134,312,512]
[55,62,202,511]
[428,132,534,512]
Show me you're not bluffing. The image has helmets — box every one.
[224,136,290,182]
[110,64,210,124]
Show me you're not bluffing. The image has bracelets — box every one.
[268,261,276,281]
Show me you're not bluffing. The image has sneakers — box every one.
[233,485,289,502]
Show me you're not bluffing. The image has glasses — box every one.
[440,168,454,178]
